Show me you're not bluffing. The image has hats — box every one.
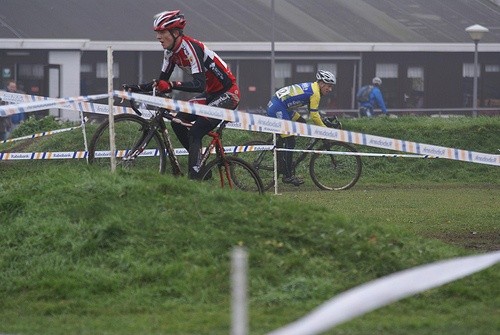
[372,77,382,85]
[316,70,336,85]
[153,9,186,31]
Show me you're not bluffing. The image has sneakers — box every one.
[282,174,306,187]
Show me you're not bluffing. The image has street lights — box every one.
[465,24,490,119]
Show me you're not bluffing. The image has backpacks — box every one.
[357,85,373,101]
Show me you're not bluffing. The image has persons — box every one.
[266,70,337,186]
[119,10,241,181]
[0,80,26,124]
[356,77,398,120]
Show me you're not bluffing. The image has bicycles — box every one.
[88,79,267,199]
[232,115,363,193]
